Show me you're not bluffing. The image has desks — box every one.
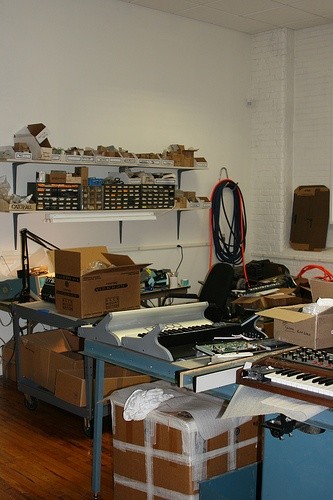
[77,340,333,500]
[14,299,109,432]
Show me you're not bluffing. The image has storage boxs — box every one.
[19,329,151,407]
[255,279,333,350]
[46,246,153,319]
[26,182,175,210]
[110,380,260,500]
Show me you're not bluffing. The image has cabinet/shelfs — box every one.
[0,158,211,251]
